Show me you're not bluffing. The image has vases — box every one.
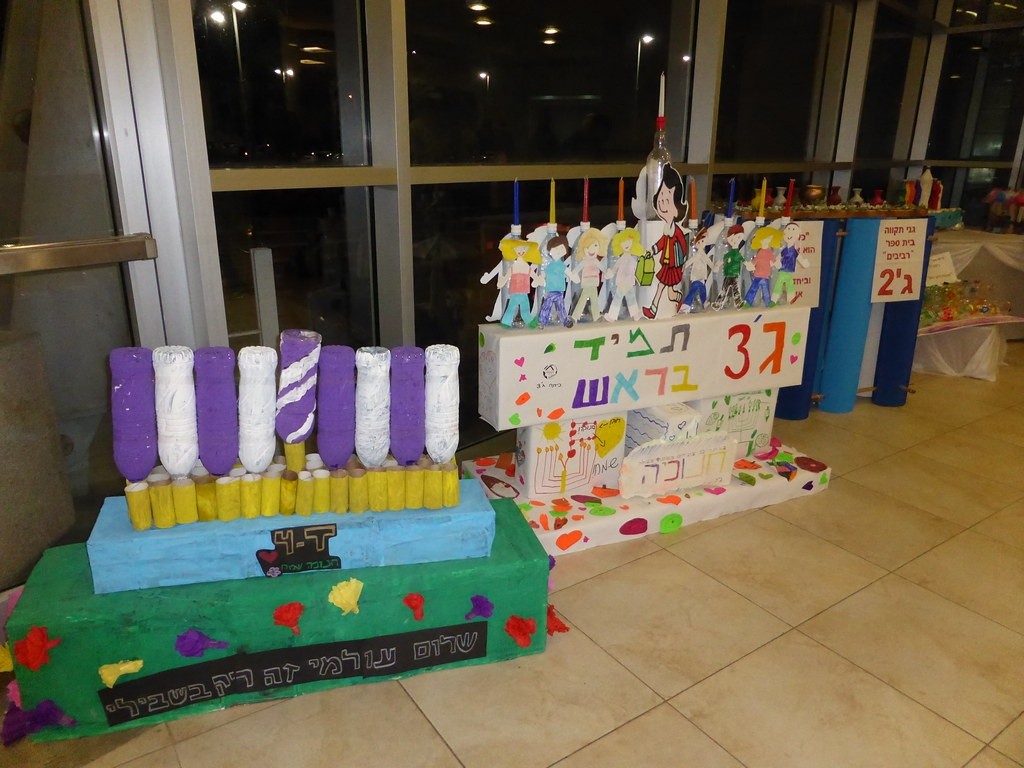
[751,184,883,212]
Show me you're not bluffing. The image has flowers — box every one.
[0,555,570,748]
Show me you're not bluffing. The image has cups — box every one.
[804,184,824,205]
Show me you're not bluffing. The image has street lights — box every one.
[232,2,248,97]
[636,34,654,91]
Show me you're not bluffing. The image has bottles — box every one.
[751,186,786,212]
[895,165,943,209]
[826,186,884,212]
[109,329,460,482]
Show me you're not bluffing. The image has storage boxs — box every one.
[478,305,811,499]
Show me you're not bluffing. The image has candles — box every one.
[583,176,589,222]
[758,177,767,217]
[514,176,519,225]
[727,178,735,217]
[658,71,665,117]
[689,176,697,219]
[785,179,794,217]
[618,177,624,220]
[549,177,556,223]
